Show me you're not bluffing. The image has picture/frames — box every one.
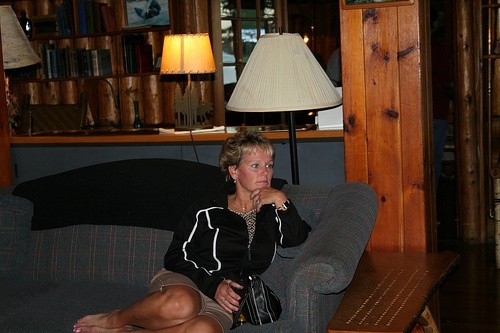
[340,0,415,10]
[122,0,173,29]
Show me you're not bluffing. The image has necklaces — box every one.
[228,198,246,210]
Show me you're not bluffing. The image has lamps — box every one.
[159,33,217,131]
[0,5,42,70]
[226,32,342,186]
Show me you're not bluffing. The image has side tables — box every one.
[327,253,462,333]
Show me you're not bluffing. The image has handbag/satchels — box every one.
[230,275,282,330]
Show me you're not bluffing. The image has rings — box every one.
[256,200,259,203]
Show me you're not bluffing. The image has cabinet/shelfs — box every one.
[8,29,161,82]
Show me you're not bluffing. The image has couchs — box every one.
[0,158,379,333]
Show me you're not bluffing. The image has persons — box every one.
[73,128,312,333]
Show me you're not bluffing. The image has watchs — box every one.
[279,198,291,210]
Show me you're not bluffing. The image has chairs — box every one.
[21,92,87,135]
